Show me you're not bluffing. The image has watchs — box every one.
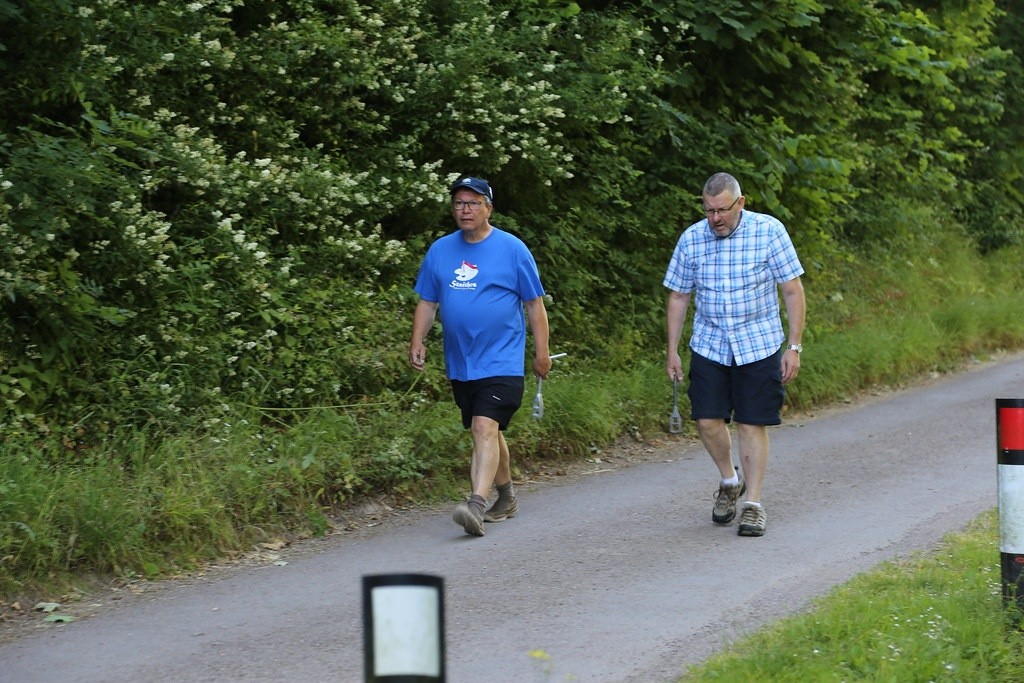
[787,344,803,353]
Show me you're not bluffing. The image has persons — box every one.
[664,173,807,537]
[410,177,553,537]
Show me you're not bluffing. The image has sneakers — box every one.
[484,489,520,523]
[711,465,747,527]
[453,503,486,537]
[737,501,768,537]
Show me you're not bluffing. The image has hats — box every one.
[449,175,491,197]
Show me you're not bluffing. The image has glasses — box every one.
[701,196,741,217]
[453,199,483,211]
